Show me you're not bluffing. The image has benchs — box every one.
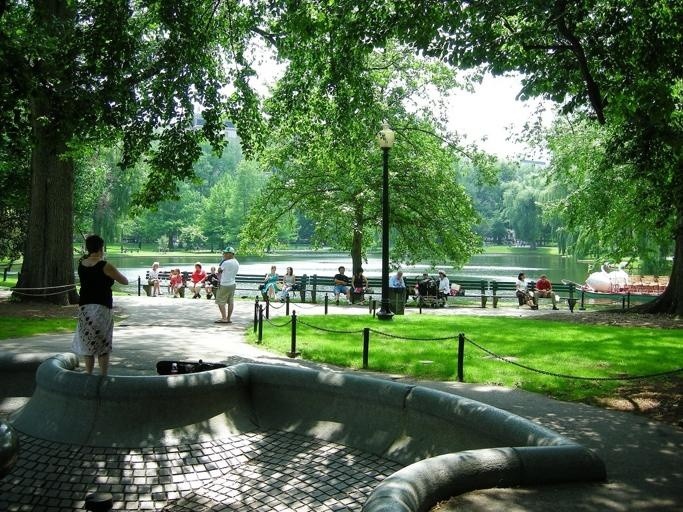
[137,270,577,314]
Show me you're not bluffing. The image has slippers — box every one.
[213,318,232,324]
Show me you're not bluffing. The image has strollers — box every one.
[414,274,440,310]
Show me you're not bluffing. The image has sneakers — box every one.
[152,291,163,297]
[335,298,368,305]
[192,293,213,299]
[530,304,559,310]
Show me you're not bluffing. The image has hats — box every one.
[193,261,202,268]
[150,261,159,268]
[438,269,446,277]
[222,246,235,255]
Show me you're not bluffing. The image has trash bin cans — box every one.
[389,287,404,315]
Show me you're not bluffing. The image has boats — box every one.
[560,275,670,307]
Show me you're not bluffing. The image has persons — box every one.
[280,267,295,303]
[351,268,368,304]
[264,266,279,301]
[389,270,406,287]
[418,272,435,296]
[215,247,239,323]
[333,267,353,306]
[516,272,538,310]
[534,275,559,310]
[168,262,219,299]
[147,262,164,297]
[71,236,128,376]
[436,270,450,307]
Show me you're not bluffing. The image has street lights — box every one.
[371,117,397,321]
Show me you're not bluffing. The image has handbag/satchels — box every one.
[449,282,465,296]
[352,287,363,294]
[291,283,302,291]
